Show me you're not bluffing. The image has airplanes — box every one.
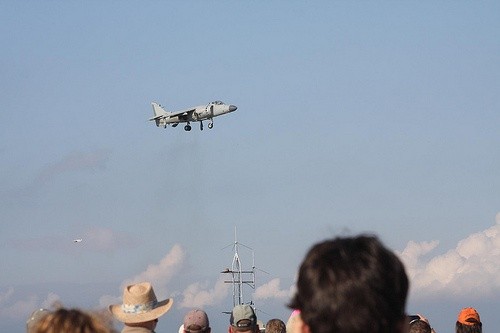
[143,100,237,131]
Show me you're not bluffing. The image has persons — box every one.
[265,319,286,333]
[184,310,211,333]
[287,233,408,333]
[228,305,260,333]
[109,282,173,333]
[408,314,435,333]
[27,308,117,333]
[456,308,482,333]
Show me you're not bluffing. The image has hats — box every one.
[265,319,286,333]
[457,308,479,326]
[179,324,184,333]
[184,309,209,333]
[109,282,174,323]
[230,305,256,327]
